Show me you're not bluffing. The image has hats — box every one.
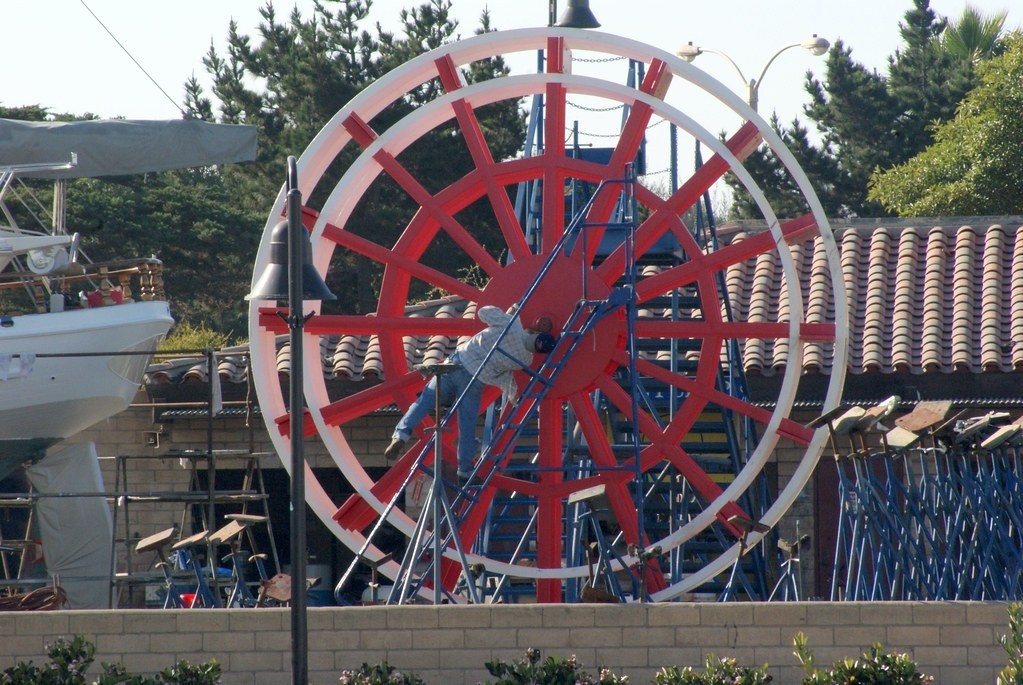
[526,332,555,354]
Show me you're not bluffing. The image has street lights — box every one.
[244,155,338,685]
[677,34,829,119]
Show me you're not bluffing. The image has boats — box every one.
[0,0,257,485]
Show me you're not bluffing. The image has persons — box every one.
[383,305,554,486]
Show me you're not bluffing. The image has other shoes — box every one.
[384,438,405,460]
[458,475,482,486]
